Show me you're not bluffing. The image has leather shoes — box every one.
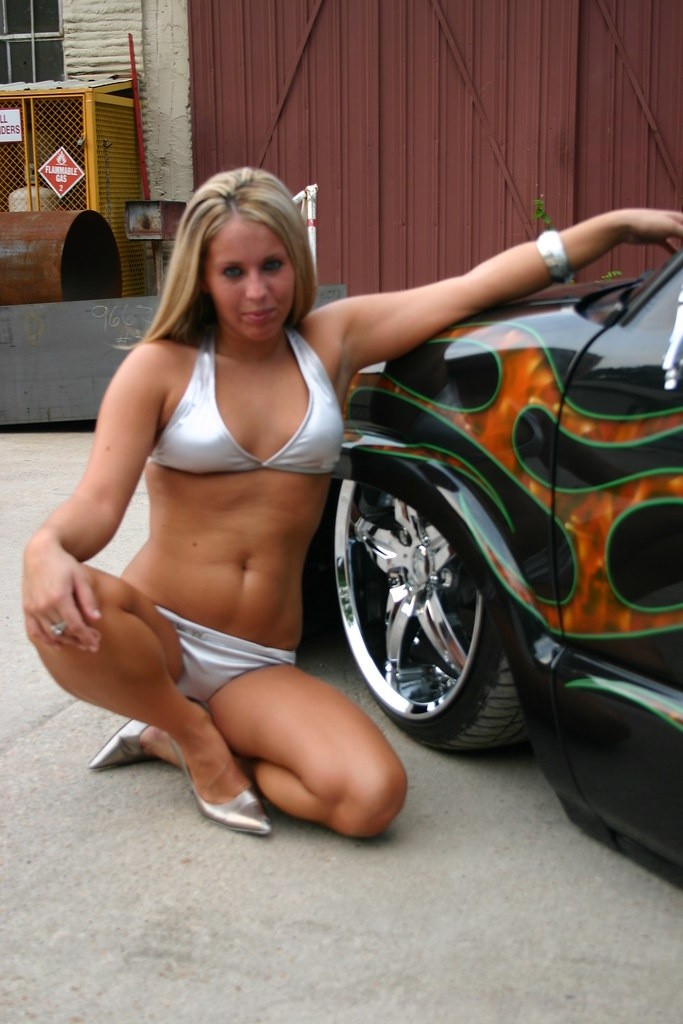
[170,692,272,834]
[88,716,153,770]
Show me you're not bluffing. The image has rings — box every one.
[50,621,65,636]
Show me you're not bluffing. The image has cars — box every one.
[311,236,683,879]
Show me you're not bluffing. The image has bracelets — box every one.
[536,231,575,284]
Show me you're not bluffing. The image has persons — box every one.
[21,166,683,838]
